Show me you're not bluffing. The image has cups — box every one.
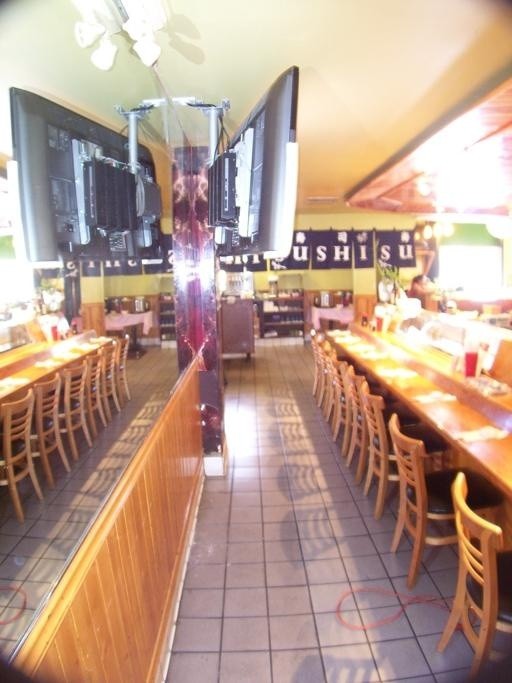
[464,344,480,376]
[372,306,404,339]
[50,324,61,342]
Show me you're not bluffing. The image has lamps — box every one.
[70,0,167,71]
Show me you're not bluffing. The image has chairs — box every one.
[0,333,130,520]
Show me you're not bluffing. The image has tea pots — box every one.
[104,295,148,317]
[314,288,352,309]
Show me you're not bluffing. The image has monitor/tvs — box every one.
[9,85,167,261]
[206,64,299,255]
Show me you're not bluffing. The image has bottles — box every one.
[254,279,305,339]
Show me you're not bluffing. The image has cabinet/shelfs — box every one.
[157,292,175,340]
[306,289,352,329]
[257,291,304,338]
